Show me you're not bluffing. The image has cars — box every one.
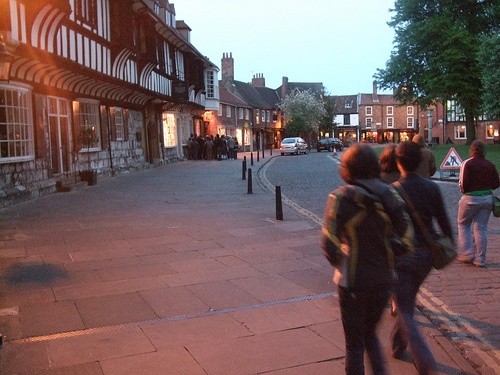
[280,137,309,155]
[316,138,345,152]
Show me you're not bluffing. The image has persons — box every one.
[379,147,402,317]
[321,142,415,375]
[457,140,500,267]
[187,134,238,160]
[386,141,456,375]
[411,134,432,179]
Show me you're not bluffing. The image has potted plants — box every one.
[79,128,98,186]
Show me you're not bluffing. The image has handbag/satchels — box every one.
[430,235,457,271]
[491,188,500,218]
[391,236,422,294]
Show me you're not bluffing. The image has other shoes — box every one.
[456,254,474,262]
[472,257,486,267]
